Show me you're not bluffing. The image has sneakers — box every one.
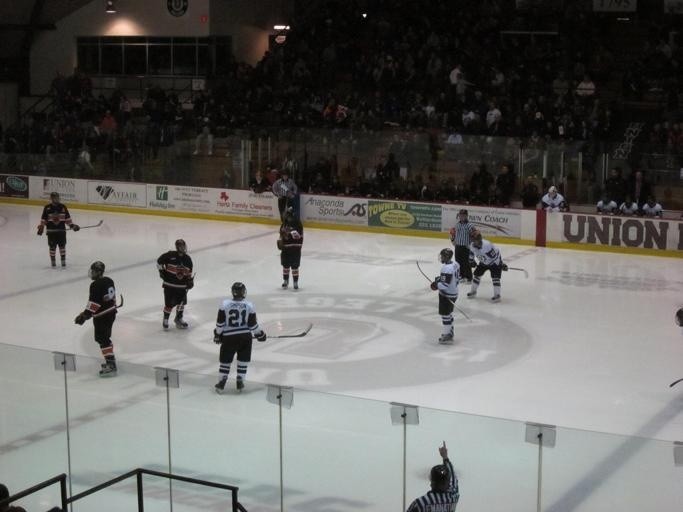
[215,379,227,390]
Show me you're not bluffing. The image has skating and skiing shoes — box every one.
[467,291,476,297]
[101,361,118,370]
[441,327,454,338]
[174,317,188,326]
[61,257,66,267]
[293,280,299,289]
[491,293,500,301]
[50,257,57,266]
[162,319,170,329]
[237,381,244,389]
[439,330,455,341]
[282,279,289,287]
[99,366,116,375]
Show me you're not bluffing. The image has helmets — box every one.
[459,209,468,215]
[675,309,683,327]
[438,248,453,265]
[471,230,483,241]
[88,261,105,279]
[288,206,298,218]
[231,283,245,297]
[50,191,59,198]
[429,465,450,492]
[174,239,188,254]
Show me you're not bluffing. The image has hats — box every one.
[549,186,558,193]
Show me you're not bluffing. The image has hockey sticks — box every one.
[173,273,196,327]
[85,294,123,321]
[43,219,103,232]
[476,264,529,279]
[416,258,470,322]
[252,323,313,339]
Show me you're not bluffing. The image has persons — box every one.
[560,200,569,212]
[596,194,618,215]
[585,172,599,204]
[36,193,79,269]
[642,196,662,219]
[631,171,649,206]
[214,284,264,394]
[467,230,507,304]
[0,484,25,512]
[559,201,569,212]
[0,67,191,183]
[520,176,541,210]
[617,196,641,217]
[450,210,475,284]
[277,207,303,289]
[544,186,564,212]
[219,166,518,209]
[74,260,117,378]
[157,239,194,331]
[606,167,626,204]
[675,307,683,326]
[431,248,460,345]
[408,442,460,512]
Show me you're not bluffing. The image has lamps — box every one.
[105,0,116,12]
